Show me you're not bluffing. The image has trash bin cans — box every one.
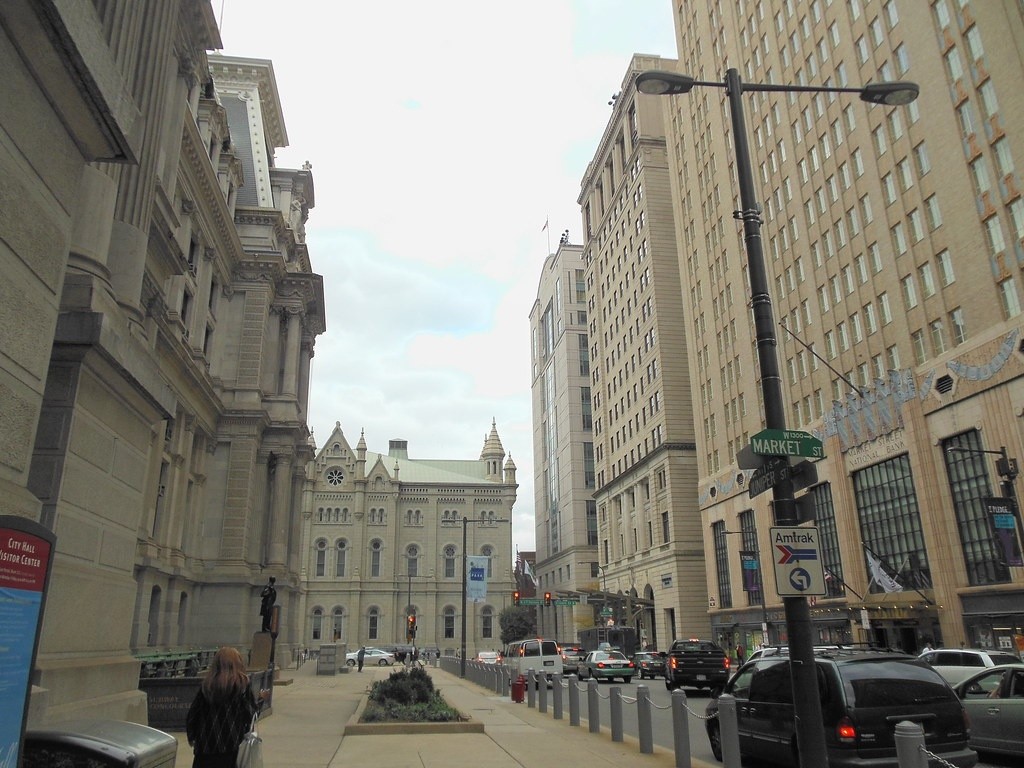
[21,717,178,768]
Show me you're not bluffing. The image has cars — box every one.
[391,645,440,660]
[345,647,395,667]
[917,647,1024,699]
[476,651,502,664]
[631,652,666,679]
[952,663,1024,757]
[561,647,634,684]
[727,644,852,690]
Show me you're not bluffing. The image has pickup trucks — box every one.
[658,639,730,691]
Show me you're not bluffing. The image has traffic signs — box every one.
[748,428,824,499]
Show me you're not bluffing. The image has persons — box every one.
[358,646,365,672]
[923,643,935,662]
[186,647,271,768]
[736,642,745,666]
[260,576,276,632]
[988,672,1006,699]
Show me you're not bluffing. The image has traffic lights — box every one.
[514,592,519,605]
[544,592,550,606]
[409,616,413,628]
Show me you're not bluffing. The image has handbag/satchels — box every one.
[235,712,263,768]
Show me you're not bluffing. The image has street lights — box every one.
[720,530,769,648]
[461,517,509,678]
[407,574,432,644]
[948,446,1024,554]
[635,68,919,767]
[578,559,610,647]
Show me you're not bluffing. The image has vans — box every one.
[502,639,563,691]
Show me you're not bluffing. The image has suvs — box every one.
[705,642,978,768]
